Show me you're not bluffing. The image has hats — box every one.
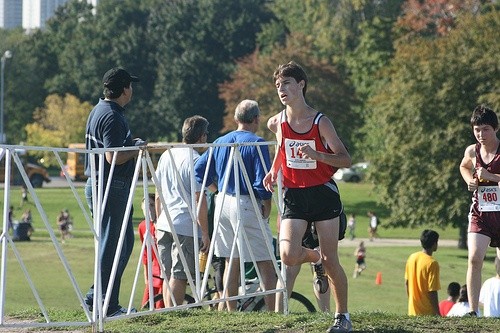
[103,67,138,92]
[448,283,460,291]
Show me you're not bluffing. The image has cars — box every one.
[333,162,378,183]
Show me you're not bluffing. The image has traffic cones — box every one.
[375,271,383,285]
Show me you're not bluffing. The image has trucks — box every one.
[138,148,165,180]
[0,149,51,188]
[61,142,88,182]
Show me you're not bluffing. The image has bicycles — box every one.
[140,274,317,313]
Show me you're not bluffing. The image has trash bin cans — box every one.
[11,222,31,241]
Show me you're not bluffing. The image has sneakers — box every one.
[326,312,353,333]
[311,246,329,294]
[97,306,137,318]
[80,295,93,311]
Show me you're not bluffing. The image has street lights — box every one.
[0,50,12,144]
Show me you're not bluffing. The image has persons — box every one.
[21,210,31,224]
[459,106,500,318]
[405,229,440,318]
[447,284,480,317]
[8,207,13,230]
[262,62,353,333]
[479,256,500,317]
[57,210,72,244]
[21,185,27,201]
[353,242,366,278]
[368,211,377,240]
[347,213,355,240]
[194,99,277,311]
[138,192,165,310]
[151,115,210,309]
[439,282,460,317]
[84,67,141,318]
